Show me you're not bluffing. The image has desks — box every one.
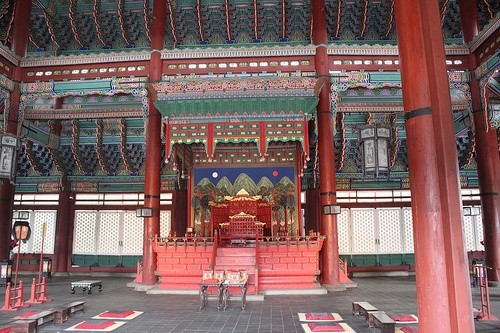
[198,279,223,309]
[222,280,249,311]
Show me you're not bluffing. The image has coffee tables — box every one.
[71,280,102,295]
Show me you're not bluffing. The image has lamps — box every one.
[472,261,486,287]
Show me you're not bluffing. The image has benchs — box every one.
[60,300,84,312]
[370,312,397,333]
[21,308,58,333]
[351,299,377,323]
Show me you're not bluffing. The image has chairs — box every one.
[218,211,266,243]
